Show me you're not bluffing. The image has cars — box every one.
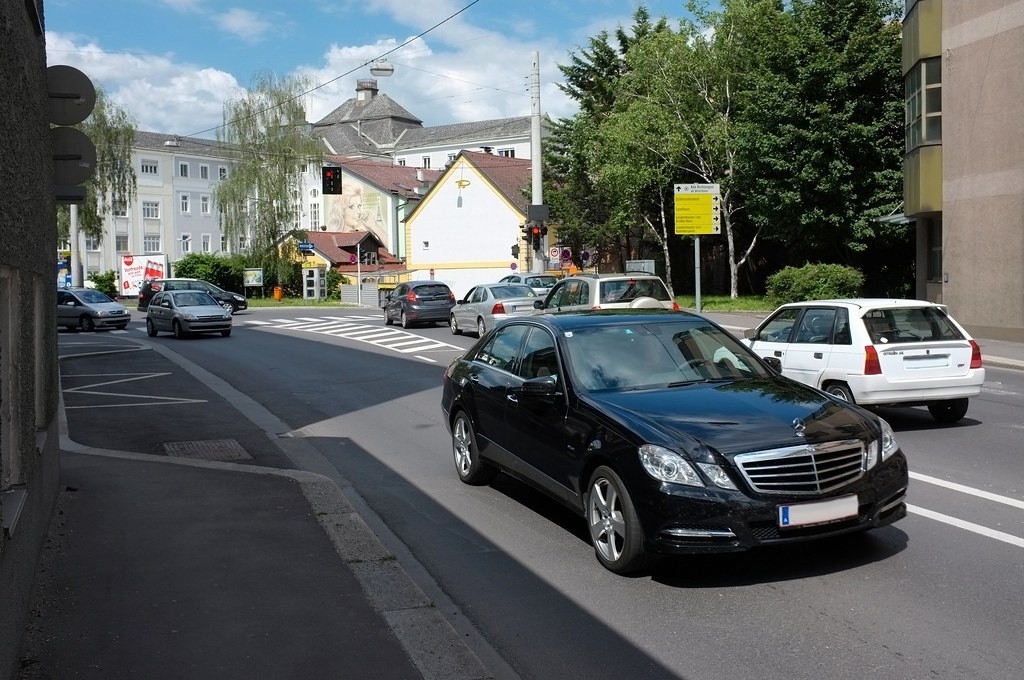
[713,298,985,426]
[146,290,232,340]
[383,280,456,329]
[447,283,544,339]
[499,272,557,300]
[441,309,910,576]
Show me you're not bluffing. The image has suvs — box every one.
[138,278,248,316]
[57,286,131,332]
[534,270,681,311]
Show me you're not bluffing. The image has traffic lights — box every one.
[520,223,532,244]
[325,170,332,193]
[541,227,548,236]
[532,226,541,251]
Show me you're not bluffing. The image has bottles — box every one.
[145,260,163,291]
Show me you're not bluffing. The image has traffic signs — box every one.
[674,184,721,234]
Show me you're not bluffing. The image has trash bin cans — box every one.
[274,287,282,302]
[379,283,398,308]
